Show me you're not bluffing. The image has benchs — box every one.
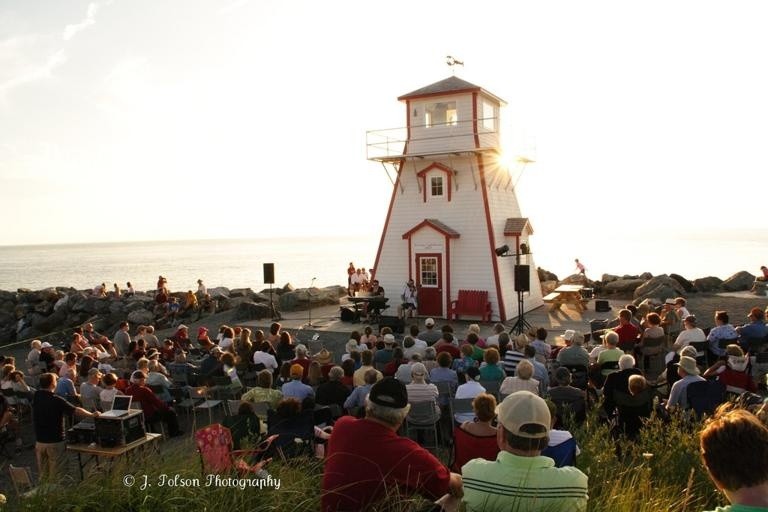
[447,289,493,324]
[542,292,560,304]
[577,294,596,312]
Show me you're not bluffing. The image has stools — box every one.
[403,305,417,324]
[367,306,392,324]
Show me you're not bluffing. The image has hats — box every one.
[499,391,550,438]
[561,329,585,345]
[131,323,243,380]
[370,376,408,408]
[290,345,378,379]
[515,334,529,350]
[467,366,480,381]
[41,341,104,377]
[383,334,394,344]
[425,318,435,327]
[663,295,764,374]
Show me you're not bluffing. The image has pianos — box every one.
[348,297,388,303]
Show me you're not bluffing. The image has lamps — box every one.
[519,243,529,254]
[494,244,509,257]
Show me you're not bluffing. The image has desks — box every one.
[548,284,588,313]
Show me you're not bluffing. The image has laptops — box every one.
[99,395,133,417]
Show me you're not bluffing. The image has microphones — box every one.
[405,281,411,287]
[312,277,316,280]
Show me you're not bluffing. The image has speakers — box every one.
[379,318,405,334]
[263,263,274,284]
[515,264,529,292]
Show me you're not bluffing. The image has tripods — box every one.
[303,295,329,330]
[516,292,533,336]
[508,292,529,336]
[258,284,280,322]
[403,295,410,327]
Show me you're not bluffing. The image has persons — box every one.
[462,390,589,511]
[321,377,464,510]
[697,403,768,511]
[3,259,768,482]
